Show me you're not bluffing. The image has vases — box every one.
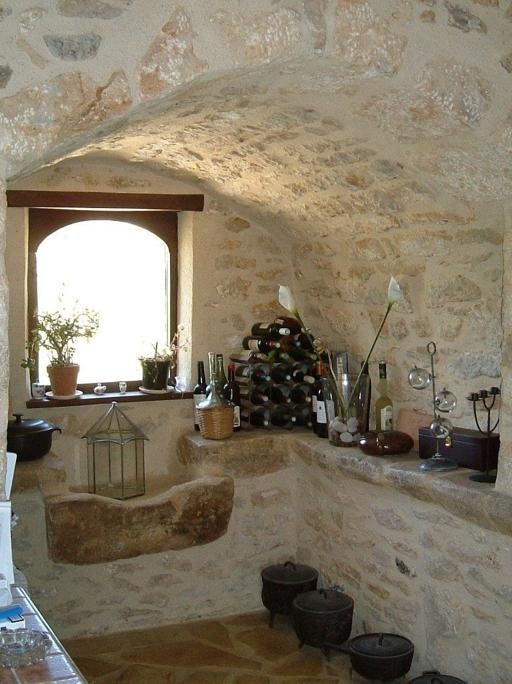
[317,373,370,447]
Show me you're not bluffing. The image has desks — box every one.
[0,584,89,684]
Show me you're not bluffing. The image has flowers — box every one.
[277,272,406,415]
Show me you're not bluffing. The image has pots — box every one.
[294,589,354,645]
[7,412,63,462]
[322,631,414,682]
[405,670,468,684]
[261,560,319,614]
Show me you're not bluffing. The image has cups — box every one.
[31,383,45,400]
[328,374,359,424]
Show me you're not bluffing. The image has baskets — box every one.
[195,404,235,441]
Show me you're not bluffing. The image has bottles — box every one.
[175,376,186,391]
[375,361,394,432]
[192,351,241,433]
[227,315,336,438]
[358,361,371,432]
[93,383,106,395]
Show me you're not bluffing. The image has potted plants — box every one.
[137,323,189,395]
[20,297,100,401]
